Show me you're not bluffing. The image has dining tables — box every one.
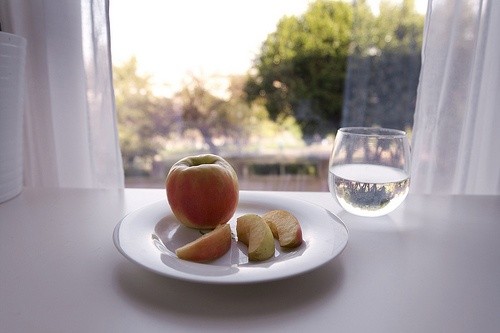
[1,186,500,333]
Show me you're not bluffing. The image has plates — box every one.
[112,190,351,284]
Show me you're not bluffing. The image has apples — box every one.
[166,154,239,229]
[262,210,302,247]
[175,224,231,263]
[237,214,276,263]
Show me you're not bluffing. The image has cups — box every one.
[329,126,415,218]
